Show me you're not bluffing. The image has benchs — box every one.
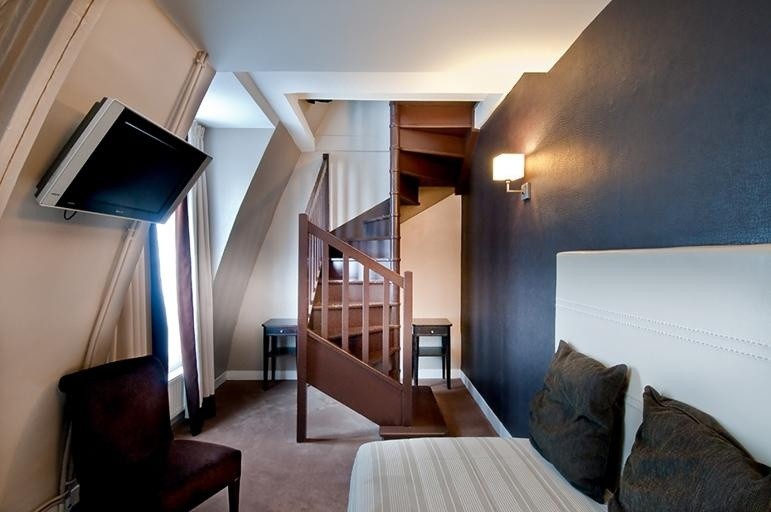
[346,243,771,511]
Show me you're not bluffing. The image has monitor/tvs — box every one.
[34,94,213,232]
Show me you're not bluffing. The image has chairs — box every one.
[58,352,244,511]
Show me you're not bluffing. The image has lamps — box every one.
[492,151,533,205]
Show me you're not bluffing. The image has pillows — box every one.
[607,380,771,511]
[528,337,633,506]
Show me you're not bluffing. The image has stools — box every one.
[263,316,298,390]
[411,317,454,391]
